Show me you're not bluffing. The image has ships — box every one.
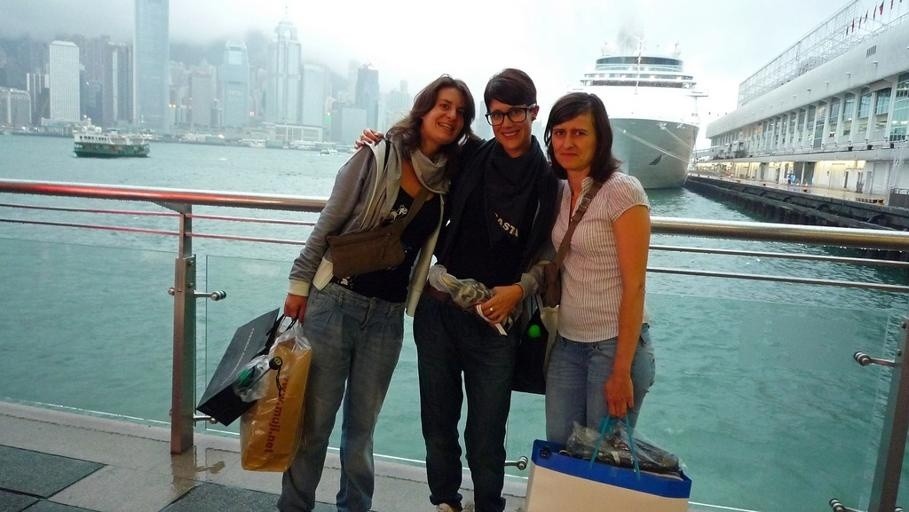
[570,46,708,188]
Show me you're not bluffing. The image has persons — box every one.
[787,175,791,189]
[544,91,655,452]
[276,74,476,512]
[356,68,557,512]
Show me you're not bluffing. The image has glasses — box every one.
[485,103,537,125]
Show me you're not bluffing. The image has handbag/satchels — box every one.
[510,297,557,394]
[523,439,692,512]
[541,264,561,308]
[197,310,299,427]
[326,224,404,277]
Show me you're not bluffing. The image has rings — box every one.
[490,307,495,312]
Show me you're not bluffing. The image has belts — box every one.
[426,284,483,320]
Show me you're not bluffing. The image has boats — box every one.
[72,129,154,157]
